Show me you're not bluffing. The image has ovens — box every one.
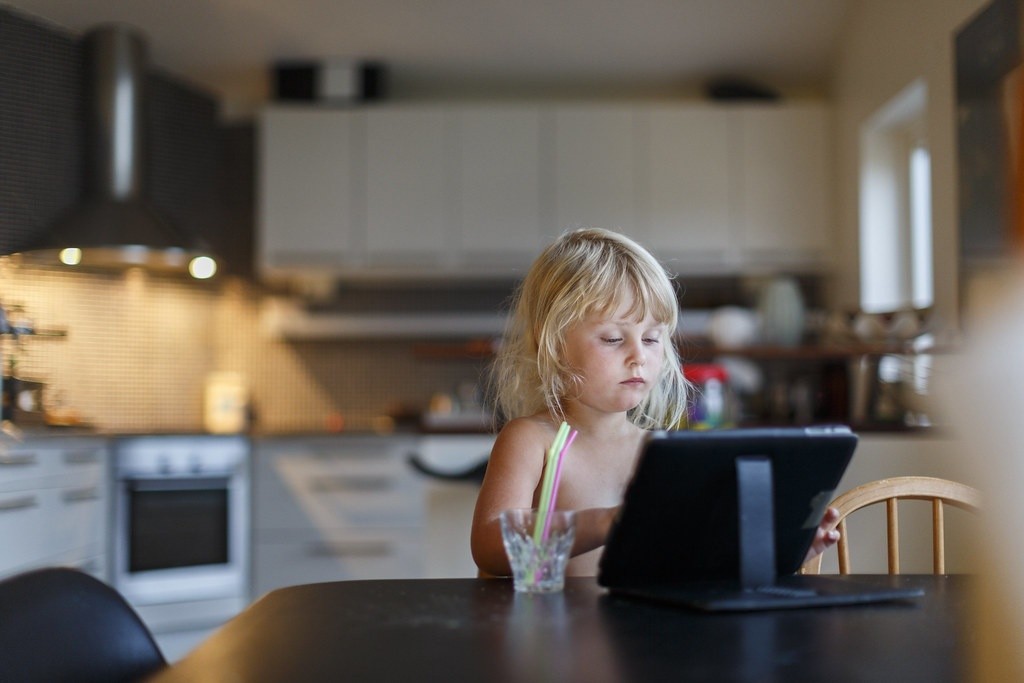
[114,444,248,605]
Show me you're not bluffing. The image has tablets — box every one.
[596,425,860,595]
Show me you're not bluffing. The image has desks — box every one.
[152,573,1024,683]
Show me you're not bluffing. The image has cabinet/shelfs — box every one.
[820,436,976,573]
[253,428,501,601]
[0,435,116,583]
[256,103,838,282]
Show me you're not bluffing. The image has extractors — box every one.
[28,205,224,283]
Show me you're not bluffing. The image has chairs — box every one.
[0,568,171,683]
[798,475,987,574]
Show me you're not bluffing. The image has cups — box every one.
[204,371,249,434]
[499,507,577,594]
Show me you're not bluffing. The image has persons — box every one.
[470,227,840,576]
